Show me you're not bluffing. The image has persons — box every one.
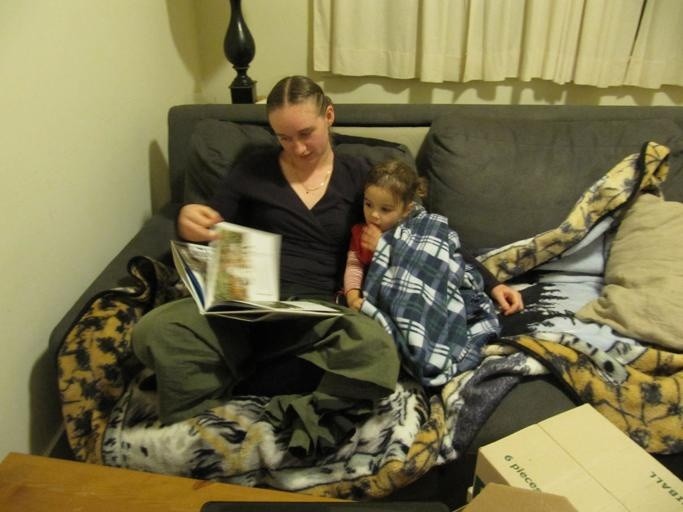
[128,75,524,457]
[342,161,431,310]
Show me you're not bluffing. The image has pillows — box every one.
[575,193,682,352]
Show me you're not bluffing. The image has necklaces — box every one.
[293,159,330,193]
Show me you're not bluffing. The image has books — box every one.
[170,221,343,322]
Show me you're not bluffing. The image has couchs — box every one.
[29,105,682,505]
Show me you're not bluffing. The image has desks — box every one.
[0,450,350,510]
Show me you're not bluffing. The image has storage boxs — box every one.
[472,401,682,512]
